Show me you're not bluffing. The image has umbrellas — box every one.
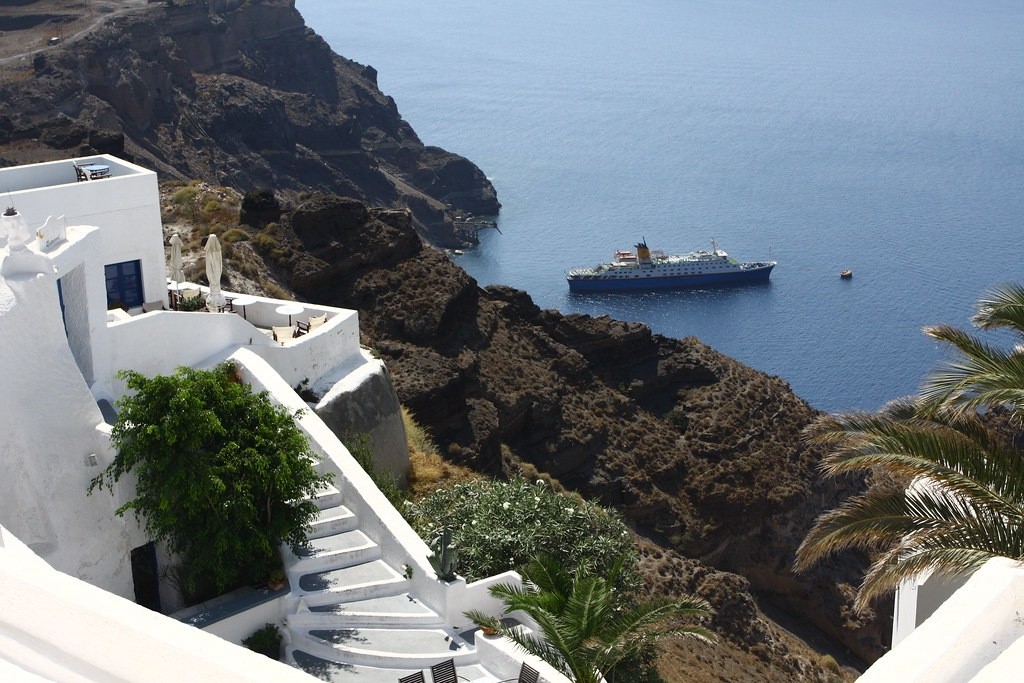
[204,233,228,313]
[169,234,185,303]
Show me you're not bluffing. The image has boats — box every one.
[565,234,776,294]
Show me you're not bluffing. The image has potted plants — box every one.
[480,616,501,635]
[2,207,20,235]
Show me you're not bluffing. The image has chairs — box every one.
[72,160,97,180]
[142,300,168,313]
[272,313,328,342]
[430,658,470,683]
[219,298,234,313]
[497,661,540,683]
[82,167,112,181]
[73,165,103,182]
[176,287,202,307]
[398,670,426,683]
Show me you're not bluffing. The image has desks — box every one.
[167,284,187,308]
[84,165,110,178]
[230,298,256,320]
[276,304,304,327]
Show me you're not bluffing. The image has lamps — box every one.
[401,565,410,576]
[85,454,98,468]
